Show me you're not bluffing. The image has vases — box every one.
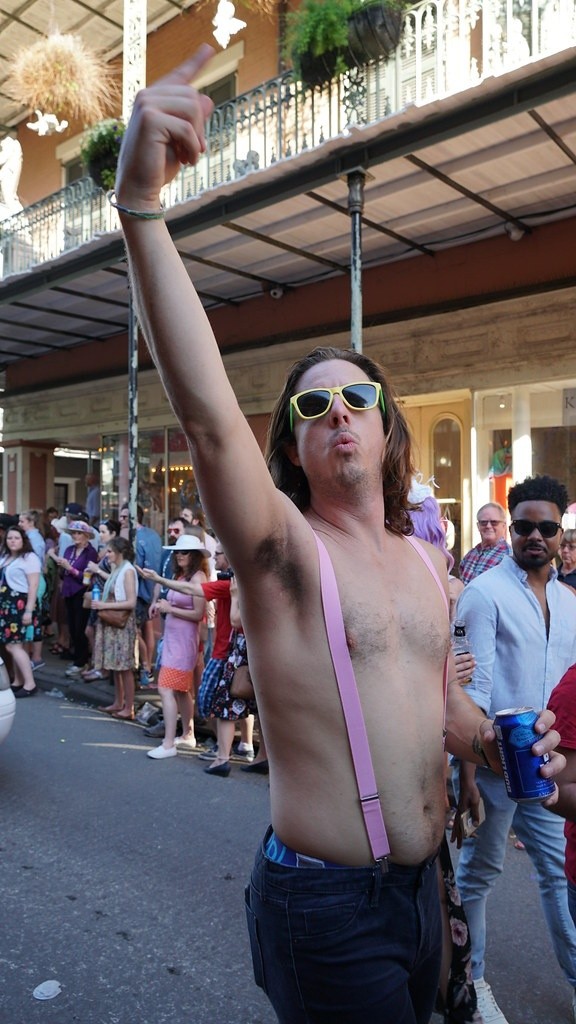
[88,143,121,192]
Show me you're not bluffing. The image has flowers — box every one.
[79,116,128,189]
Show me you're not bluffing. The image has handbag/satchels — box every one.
[97,595,130,628]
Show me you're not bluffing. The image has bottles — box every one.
[452,620,473,686]
[92,584,100,601]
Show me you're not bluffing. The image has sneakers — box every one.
[468,979,507,1024]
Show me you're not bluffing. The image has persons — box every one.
[450,474,576,1024]
[557,528,576,589]
[0,502,269,778]
[83,473,100,527]
[113,43,567,1024]
[459,502,513,588]
[540,662,576,926]
[406,477,465,616]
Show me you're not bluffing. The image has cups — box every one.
[83,572,92,585]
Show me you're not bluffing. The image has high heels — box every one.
[241,760,269,776]
[205,763,231,777]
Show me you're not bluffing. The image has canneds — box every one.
[491,705,557,803]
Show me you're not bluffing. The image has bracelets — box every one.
[108,189,167,220]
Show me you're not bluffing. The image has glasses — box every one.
[480,519,504,528]
[166,528,184,535]
[120,514,128,519]
[215,551,225,556]
[511,518,564,538]
[289,381,385,435]
[171,548,190,555]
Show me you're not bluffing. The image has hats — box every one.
[161,535,212,559]
[51,517,68,533]
[65,502,82,515]
[62,522,97,540]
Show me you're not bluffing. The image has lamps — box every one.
[498,398,507,409]
[54,449,100,462]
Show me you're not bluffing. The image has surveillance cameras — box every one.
[271,288,284,299]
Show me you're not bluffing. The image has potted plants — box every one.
[274,0,407,91]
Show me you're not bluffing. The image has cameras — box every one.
[217,567,235,580]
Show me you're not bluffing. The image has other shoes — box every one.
[51,641,255,763]
[31,658,46,672]
[9,682,37,699]
[514,841,526,849]
